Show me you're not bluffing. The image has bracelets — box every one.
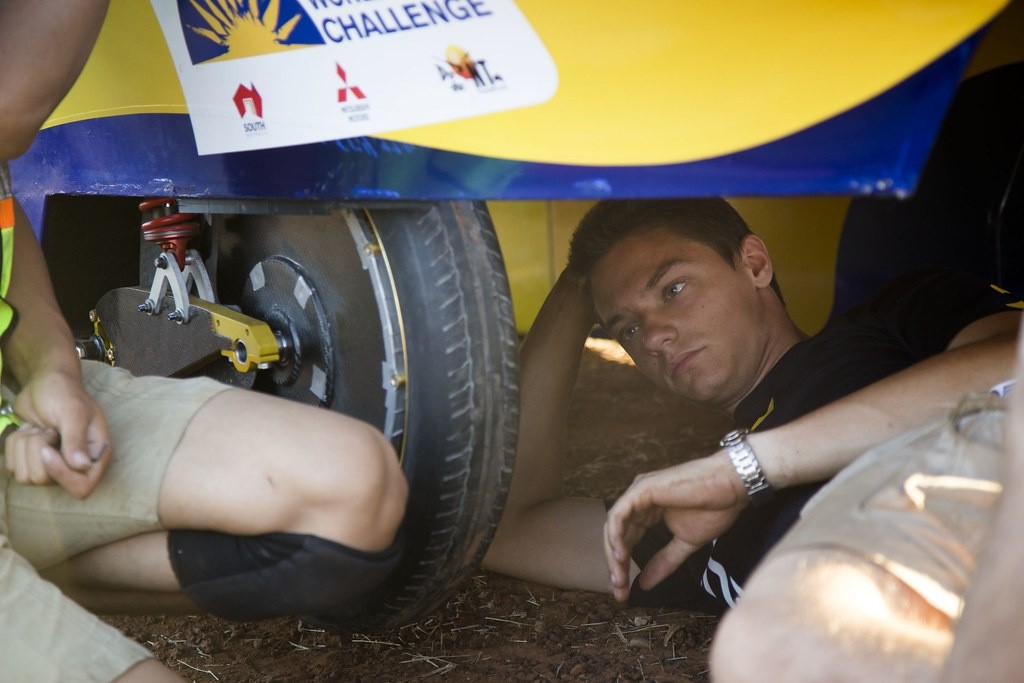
[718,430,770,500]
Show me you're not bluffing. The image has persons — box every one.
[0,0,411,683]
[480,194,1024,683]
